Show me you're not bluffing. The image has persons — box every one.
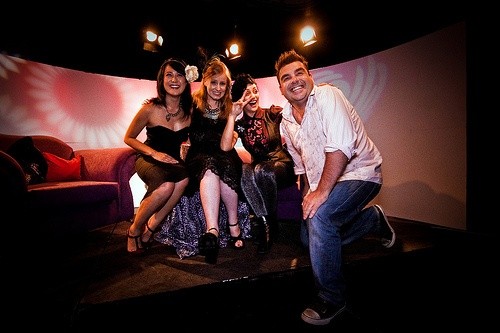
[141,56,243,264]
[218,72,334,255]
[124,58,197,258]
[273,50,396,327]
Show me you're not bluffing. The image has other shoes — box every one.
[261,222,273,251]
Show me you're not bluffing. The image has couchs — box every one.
[0,134,141,249]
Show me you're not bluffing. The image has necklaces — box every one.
[164,104,183,122]
[205,105,221,119]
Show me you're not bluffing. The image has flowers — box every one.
[185,65,200,83]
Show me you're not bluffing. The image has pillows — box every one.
[43,151,81,181]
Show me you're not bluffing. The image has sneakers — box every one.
[374,204,396,248]
[301,296,347,325]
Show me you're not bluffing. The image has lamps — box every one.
[144,29,165,51]
[225,40,242,61]
[298,23,320,47]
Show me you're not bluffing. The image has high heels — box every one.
[141,223,155,250]
[198,228,220,264]
[228,222,245,250]
[126,230,141,257]
